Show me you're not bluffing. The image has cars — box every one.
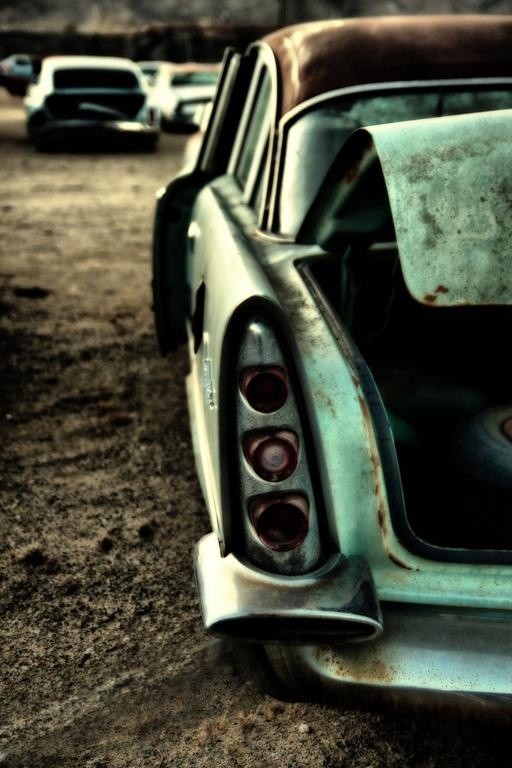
[146,14,512,716]
[0,49,227,154]
